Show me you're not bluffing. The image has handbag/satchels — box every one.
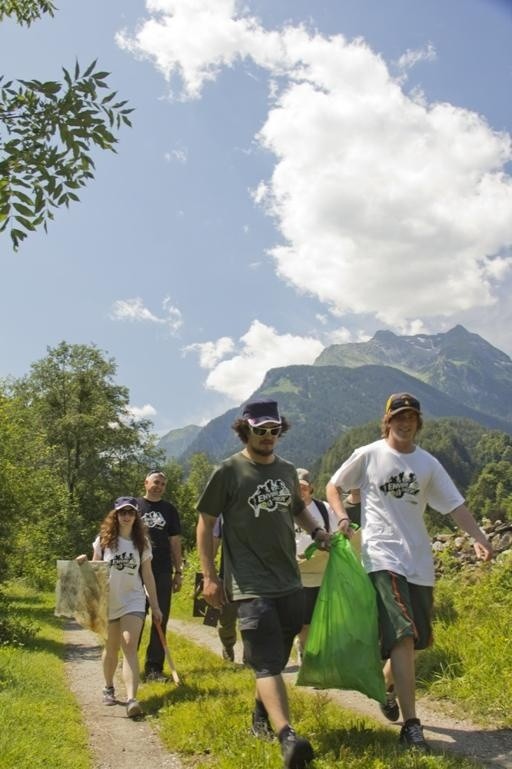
[337,516,354,526]
[174,567,184,577]
[309,526,324,540]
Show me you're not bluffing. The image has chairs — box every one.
[381,692,398,721]
[127,699,141,716]
[223,644,234,661]
[103,687,115,704]
[400,718,431,753]
[145,671,172,683]
[251,707,268,735]
[276,725,312,768]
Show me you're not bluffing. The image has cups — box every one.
[118,509,134,516]
[249,426,281,437]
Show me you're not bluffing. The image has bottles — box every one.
[386,393,420,415]
[115,497,138,510]
[243,402,282,427]
[296,468,312,486]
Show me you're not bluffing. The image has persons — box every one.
[194,401,335,768]
[75,495,163,718]
[207,511,252,669]
[323,391,496,755]
[135,470,184,685]
[287,467,340,670]
